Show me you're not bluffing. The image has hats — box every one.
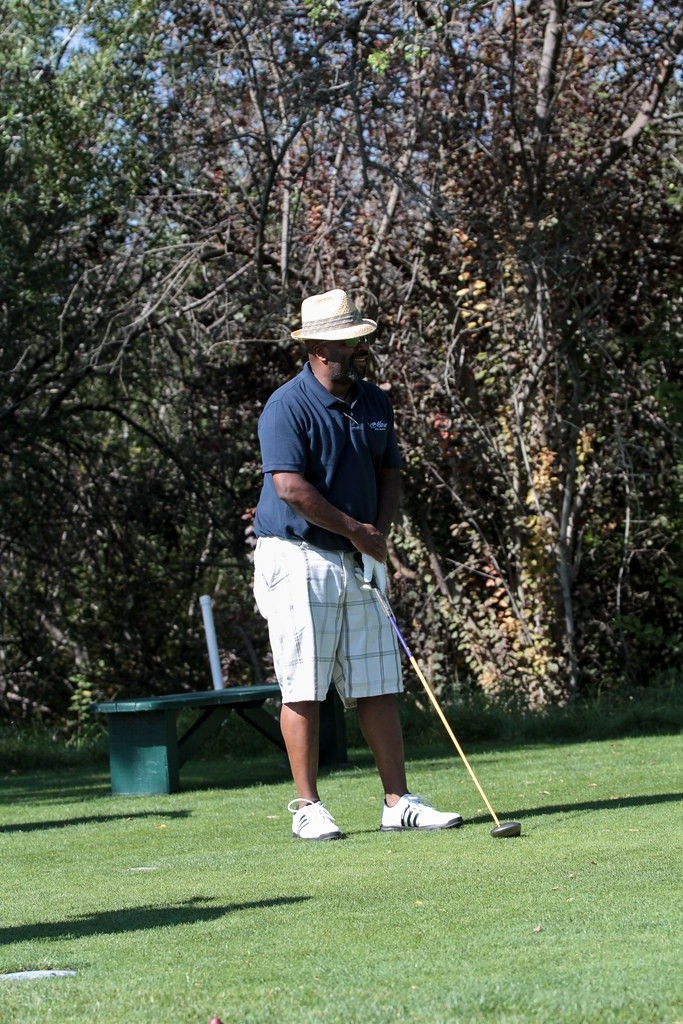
[290,289,377,341]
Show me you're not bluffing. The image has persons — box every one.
[254,289,464,839]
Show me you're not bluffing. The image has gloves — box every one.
[355,552,387,596]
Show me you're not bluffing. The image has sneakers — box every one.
[291,800,343,839]
[379,792,464,830]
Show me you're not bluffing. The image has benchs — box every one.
[95,683,350,797]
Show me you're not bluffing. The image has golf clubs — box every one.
[354,553,523,838]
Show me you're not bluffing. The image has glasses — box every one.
[318,334,370,349]
